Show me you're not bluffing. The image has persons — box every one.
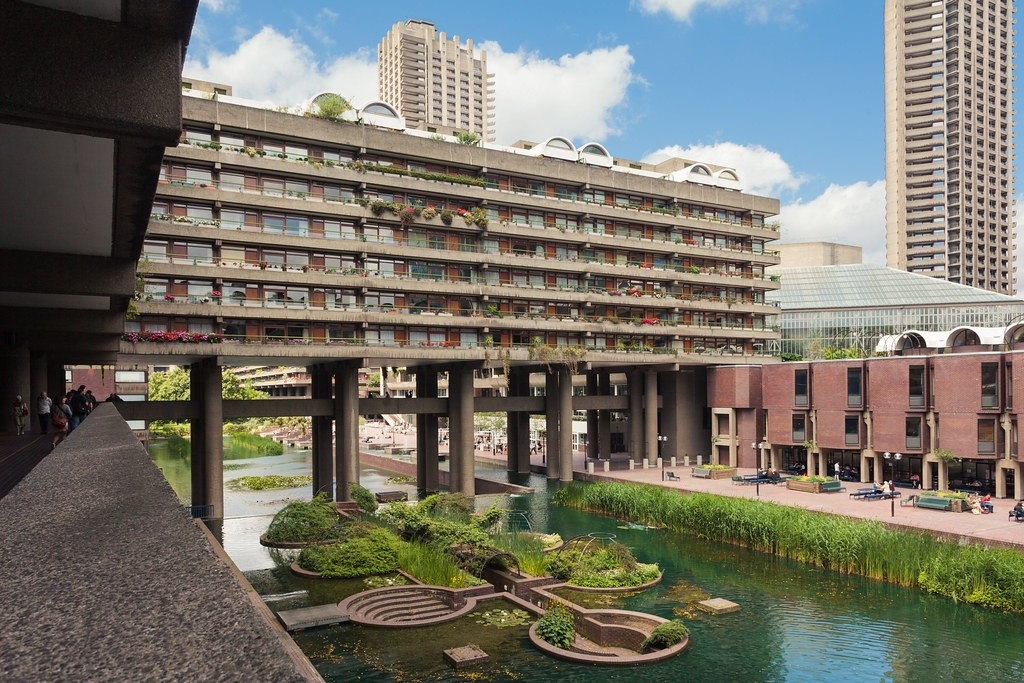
[834,461,841,483]
[104,391,123,404]
[8,383,102,451]
[874,480,895,500]
[1014,502,1024,520]
[791,461,806,476]
[966,492,993,515]
[759,468,780,485]
[910,473,920,488]
[530,438,542,455]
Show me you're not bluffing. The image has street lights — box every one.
[657,434,668,481]
[882,451,902,517]
[751,441,764,496]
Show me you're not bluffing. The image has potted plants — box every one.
[615,341,627,353]
[916,448,972,513]
[261,498,343,567]
[771,324,781,332]
[786,440,835,494]
[486,304,497,318]
[498,311,504,318]
[493,531,563,554]
[588,346,606,352]
[642,343,653,354]
[693,433,738,481]
[770,274,782,281]
[629,343,640,353]
[290,522,399,603]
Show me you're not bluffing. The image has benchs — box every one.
[864,492,902,501]
[849,491,874,501]
[732,476,743,486]
[665,471,680,482]
[916,496,953,513]
[749,479,772,485]
[1009,514,1024,522]
[689,467,711,479]
[900,494,914,507]
[821,480,847,494]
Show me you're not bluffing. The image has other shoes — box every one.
[17,433,20,435]
[51,443,56,450]
[21,432,24,435]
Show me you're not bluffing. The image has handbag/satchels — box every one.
[51,416,67,428]
[22,407,29,416]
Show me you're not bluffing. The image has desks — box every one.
[857,487,877,501]
